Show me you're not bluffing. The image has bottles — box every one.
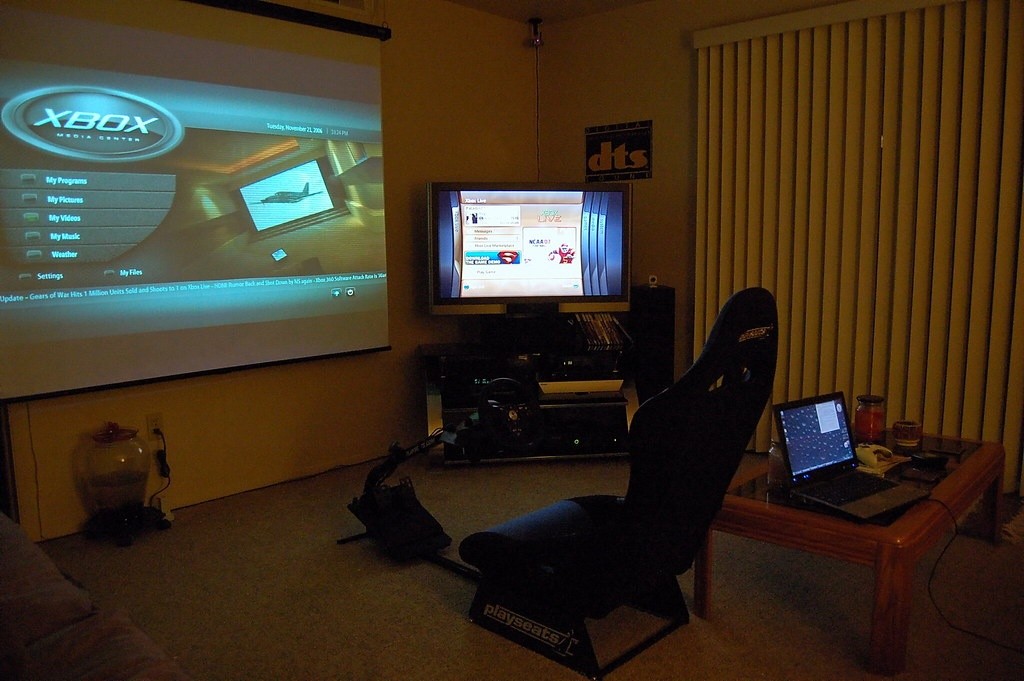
[84,422,151,514]
[855,395,885,440]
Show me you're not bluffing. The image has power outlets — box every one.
[147,411,166,440]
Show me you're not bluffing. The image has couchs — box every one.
[0,512,190,681]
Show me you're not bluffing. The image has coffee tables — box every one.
[690,427,1006,678]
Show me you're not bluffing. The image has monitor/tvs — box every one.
[425,183,631,316]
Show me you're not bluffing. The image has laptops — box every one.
[770,390,930,524]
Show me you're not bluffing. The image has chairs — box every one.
[458,288,778,681]
